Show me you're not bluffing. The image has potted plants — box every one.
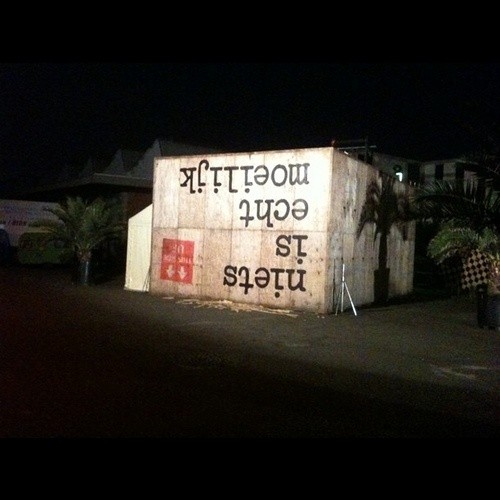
[17,197,127,289]
